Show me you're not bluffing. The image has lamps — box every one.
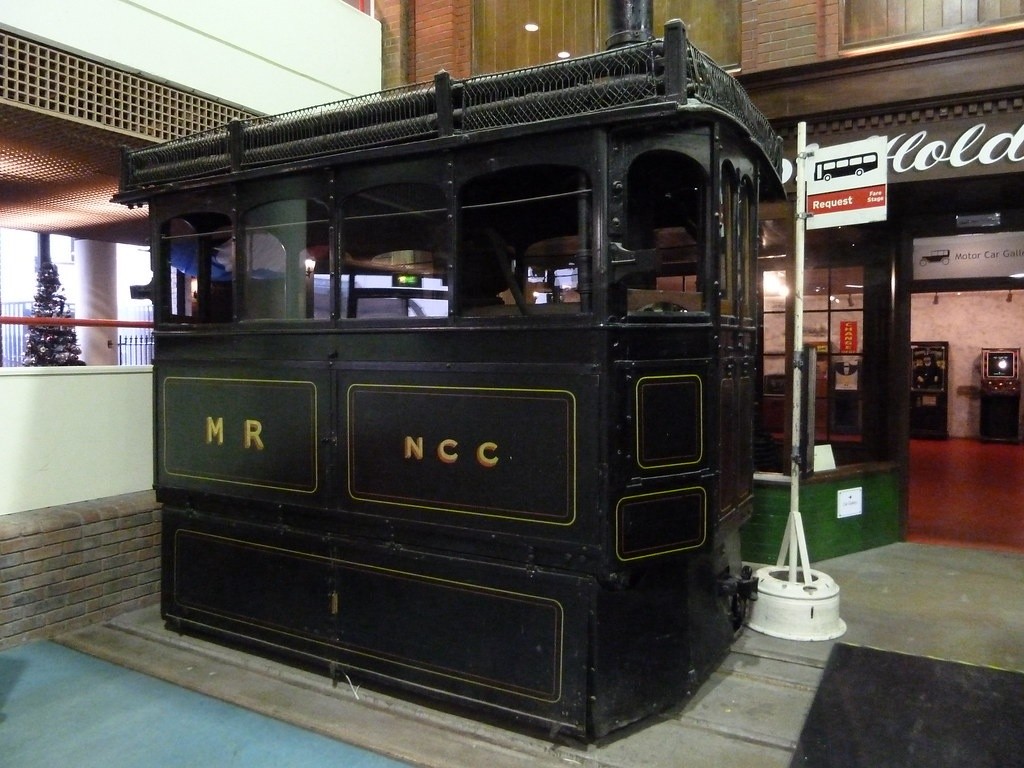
[847,293,854,307]
[932,293,938,304]
[1006,289,1013,303]
